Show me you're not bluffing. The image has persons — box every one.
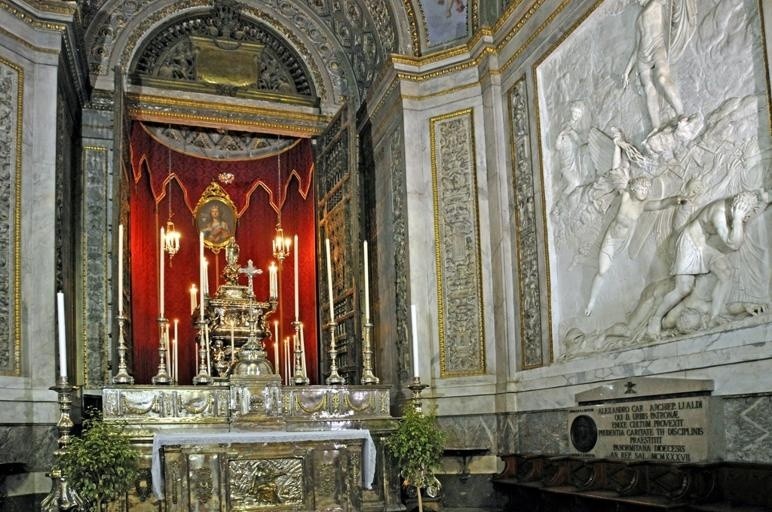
[207,244,267,378]
[199,202,233,245]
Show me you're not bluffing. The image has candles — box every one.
[57,289,68,377]
[326,238,335,323]
[159,226,166,316]
[120,223,123,312]
[294,232,299,322]
[364,240,371,324]
[411,304,421,378]
[169,230,295,386]
